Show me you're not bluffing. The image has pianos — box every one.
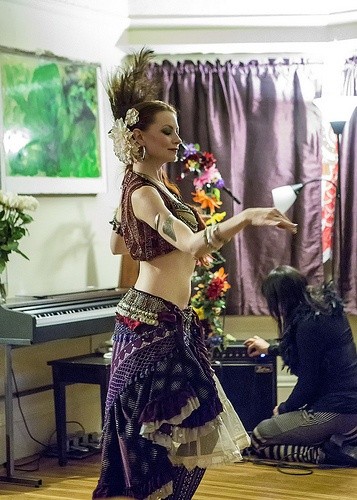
[1,284,133,488]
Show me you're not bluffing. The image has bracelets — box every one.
[203,226,221,253]
[212,222,232,246]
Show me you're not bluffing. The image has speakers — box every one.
[210,345,277,433]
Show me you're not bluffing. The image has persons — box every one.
[91,45,299,500]
[242,264,357,467]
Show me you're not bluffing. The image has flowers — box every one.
[107,108,139,167]
[0,189,38,303]
[182,141,231,349]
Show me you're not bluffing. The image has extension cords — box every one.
[65,432,99,453]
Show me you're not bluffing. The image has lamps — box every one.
[271,94,357,299]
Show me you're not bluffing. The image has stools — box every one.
[47,352,112,466]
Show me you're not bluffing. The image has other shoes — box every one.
[322,446,357,465]
